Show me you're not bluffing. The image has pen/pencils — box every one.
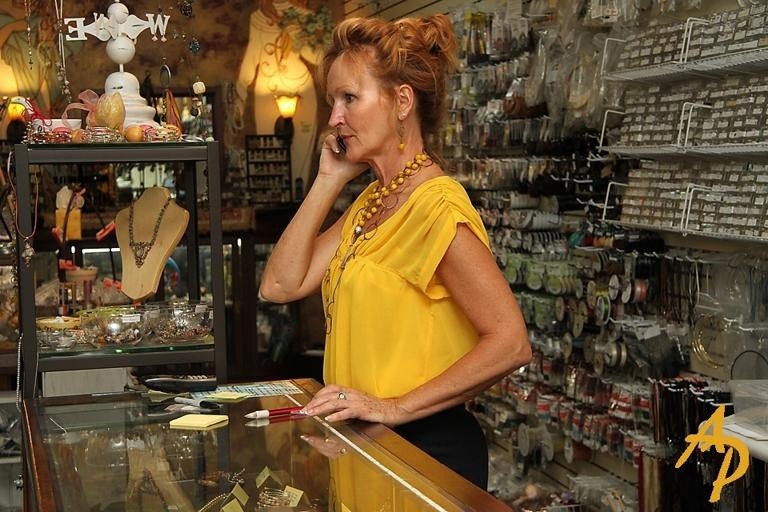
[174,396,220,409]
[244,406,304,419]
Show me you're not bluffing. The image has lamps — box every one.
[274,94,298,141]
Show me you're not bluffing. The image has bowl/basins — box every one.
[44,301,217,356]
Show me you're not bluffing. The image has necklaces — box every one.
[128,196,172,267]
[323,154,434,335]
[7,150,39,268]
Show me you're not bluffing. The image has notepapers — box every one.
[170,414,229,428]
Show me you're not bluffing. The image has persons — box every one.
[259,13,533,493]
[301,423,465,512]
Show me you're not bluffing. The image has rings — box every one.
[338,445,349,456]
[338,392,346,400]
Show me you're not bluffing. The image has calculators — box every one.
[142,374,216,393]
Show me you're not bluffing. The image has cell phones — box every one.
[336,135,346,152]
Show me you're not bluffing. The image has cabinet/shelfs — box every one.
[12,137,221,399]
[448,0,767,512]
